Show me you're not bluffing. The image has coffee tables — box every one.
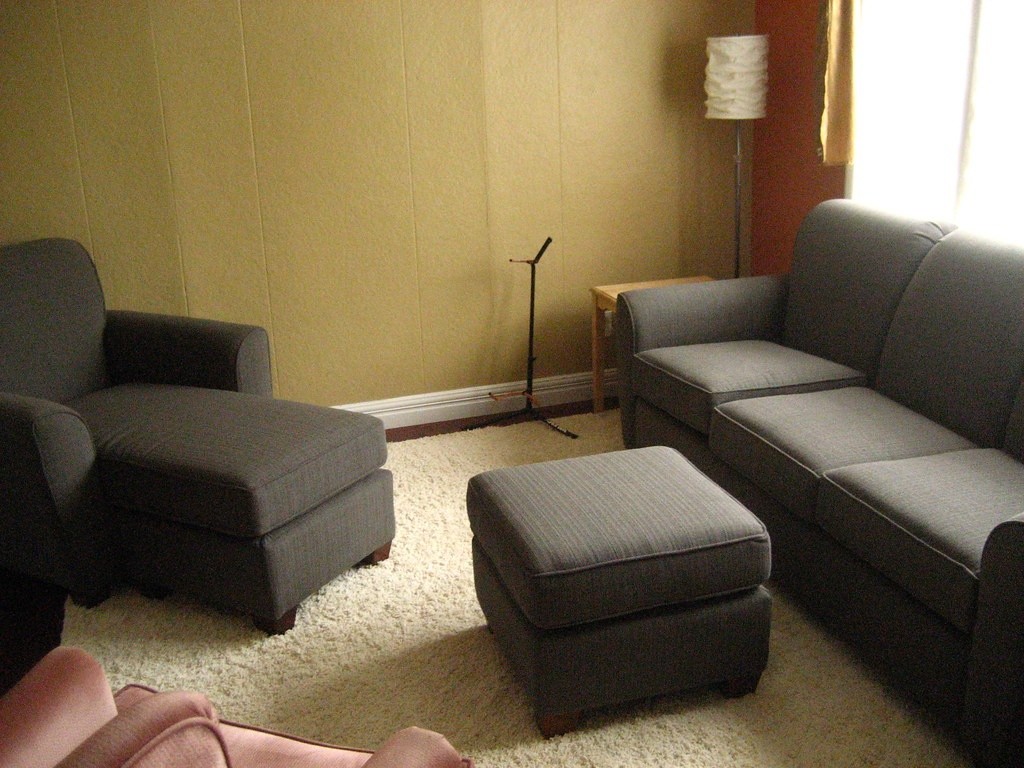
[589,275,717,416]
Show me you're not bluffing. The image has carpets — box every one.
[57,406,977,768]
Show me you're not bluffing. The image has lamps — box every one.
[705,33,770,275]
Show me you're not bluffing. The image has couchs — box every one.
[0,235,396,635]
[0,644,475,768]
[617,199,1024,768]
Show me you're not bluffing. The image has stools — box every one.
[466,445,773,740]
